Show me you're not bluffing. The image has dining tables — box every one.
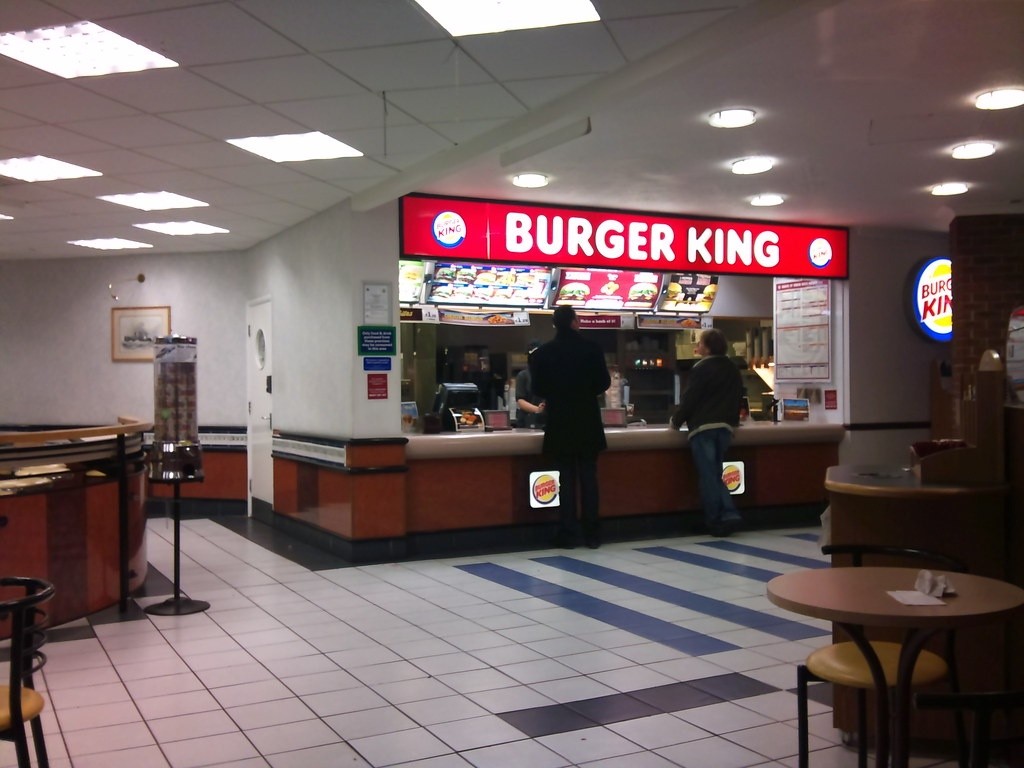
[767,568,1024,768]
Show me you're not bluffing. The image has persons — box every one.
[528,305,613,551]
[433,343,506,416]
[515,342,547,430]
[669,329,744,537]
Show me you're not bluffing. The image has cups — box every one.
[503,378,516,421]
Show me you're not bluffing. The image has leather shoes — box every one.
[692,517,745,537]
[545,532,602,550]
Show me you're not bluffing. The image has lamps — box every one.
[108,273,145,302]
[141,441,210,615]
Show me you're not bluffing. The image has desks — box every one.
[824,461,1010,744]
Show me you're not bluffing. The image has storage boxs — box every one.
[909,439,979,483]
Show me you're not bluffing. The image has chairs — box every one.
[914,691,1024,767]
[795,543,972,768]
[0,575,56,768]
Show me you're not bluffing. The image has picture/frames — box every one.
[363,357,392,371]
[357,325,397,355]
[367,374,388,399]
[111,306,171,362]
[363,279,393,327]
